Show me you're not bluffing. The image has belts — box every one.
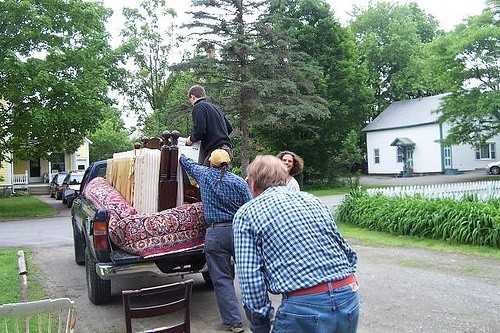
[282,275,355,296]
[209,221,232,226]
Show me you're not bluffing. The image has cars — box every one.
[62,170,87,208]
[485,162,499,176]
[50,172,69,201]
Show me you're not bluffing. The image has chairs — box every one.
[122,279,194,333]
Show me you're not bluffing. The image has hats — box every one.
[209,150,230,166]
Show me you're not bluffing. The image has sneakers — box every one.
[221,321,244,332]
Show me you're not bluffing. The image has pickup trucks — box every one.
[71,160,235,305]
